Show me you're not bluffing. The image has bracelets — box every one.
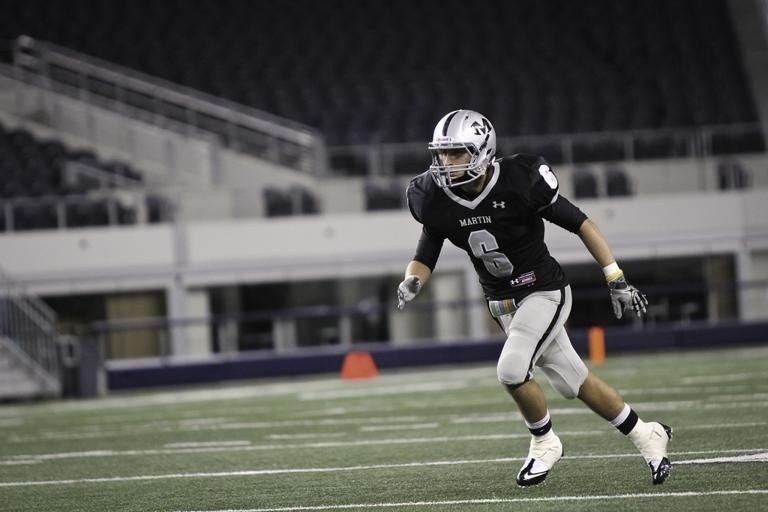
[601,262,621,276]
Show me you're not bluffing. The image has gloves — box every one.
[602,260,649,320]
[396,274,423,311]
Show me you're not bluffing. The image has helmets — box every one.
[427,108,497,175]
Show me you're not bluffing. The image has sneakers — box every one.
[635,419,673,486]
[515,435,564,489]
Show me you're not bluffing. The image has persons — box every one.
[396,109,672,487]
